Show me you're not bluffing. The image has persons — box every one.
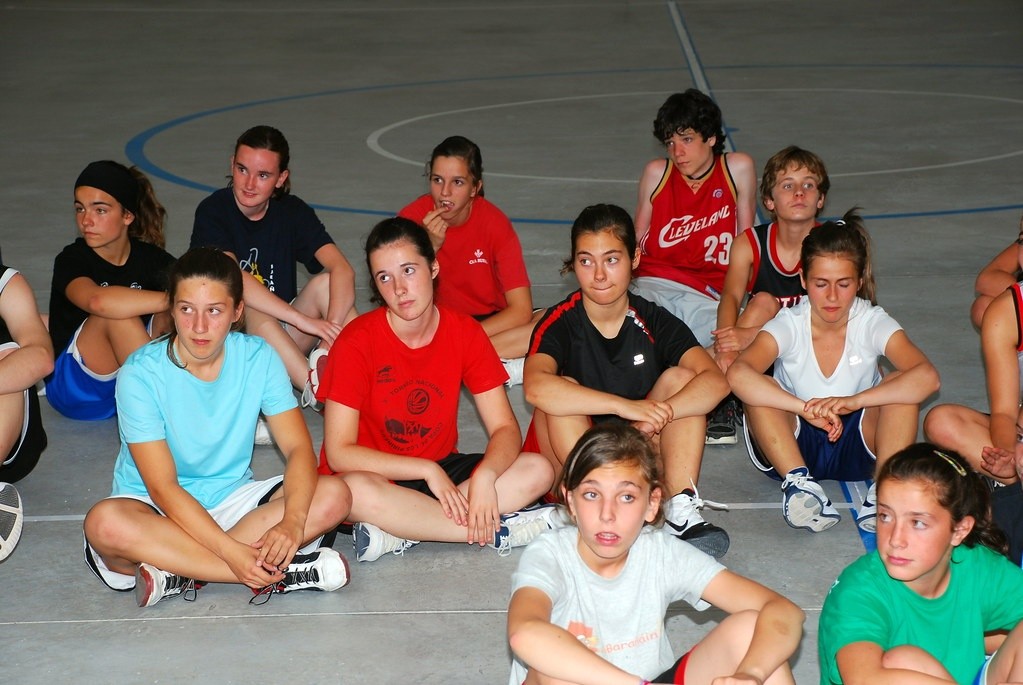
[189,123,359,409]
[0,246,55,563]
[46,160,182,423]
[817,443,1023,685]
[925,213,1022,523]
[524,203,731,559]
[507,424,806,684]
[727,205,942,533]
[312,216,555,564]
[626,87,759,444]
[399,135,553,387]
[713,146,840,421]
[83,249,353,608]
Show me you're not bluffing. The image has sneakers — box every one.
[498,356,524,387]
[301,348,330,412]
[254,414,275,445]
[856,482,878,533]
[704,392,742,444]
[135,562,208,607]
[353,521,419,562]
[656,479,729,559]
[0,482,23,562]
[253,547,350,592]
[488,503,570,556]
[781,466,841,533]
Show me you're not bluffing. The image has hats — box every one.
[74,160,137,216]
